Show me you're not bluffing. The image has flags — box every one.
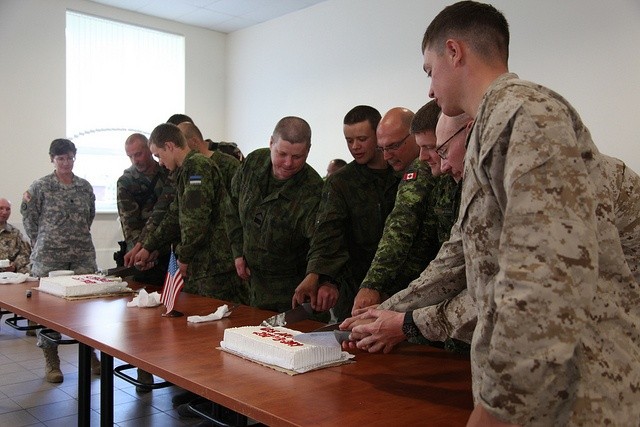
[159,249,185,317]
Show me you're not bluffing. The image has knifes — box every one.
[105,260,158,277]
[260,302,317,327]
[293,329,359,348]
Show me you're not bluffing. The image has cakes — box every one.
[39,273,132,296]
[220,325,341,370]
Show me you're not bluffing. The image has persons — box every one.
[116,134,161,391]
[325,160,346,179]
[135,121,239,269]
[376,107,419,183]
[351,112,639,355]
[124,114,244,414]
[225,116,340,313]
[147,123,252,305]
[0,197,37,336]
[339,1,639,426]
[292,106,402,312]
[352,98,457,311]
[20,139,100,382]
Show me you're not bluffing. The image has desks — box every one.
[1,277,474,425]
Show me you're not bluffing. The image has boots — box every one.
[136,368,154,393]
[91,352,101,375]
[43,344,63,383]
[172,391,200,403]
[176,397,215,417]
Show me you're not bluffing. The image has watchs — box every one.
[401,311,420,339]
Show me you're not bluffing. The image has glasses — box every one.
[376,134,410,152]
[53,156,76,162]
[435,123,467,160]
